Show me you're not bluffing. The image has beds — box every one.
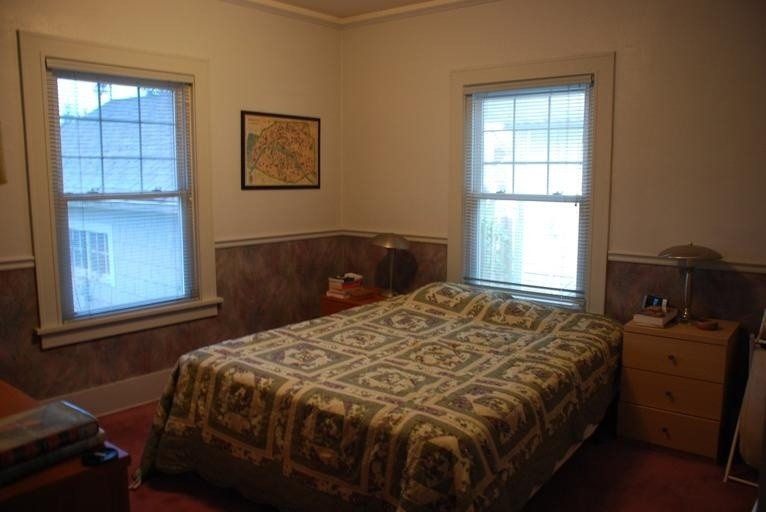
[151,280,623,512]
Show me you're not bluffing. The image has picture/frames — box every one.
[239,110,323,191]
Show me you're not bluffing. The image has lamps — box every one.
[369,231,411,299]
[657,238,724,325]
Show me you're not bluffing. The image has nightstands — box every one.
[620,308,744,463]
[319,286,390,317]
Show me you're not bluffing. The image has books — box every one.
[0,426,106,486]
[0,399,99,467]
[632,304,678,326]
[326,274,375,300]
[636,322,664,328]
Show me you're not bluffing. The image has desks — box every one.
[1,376,132,512]
